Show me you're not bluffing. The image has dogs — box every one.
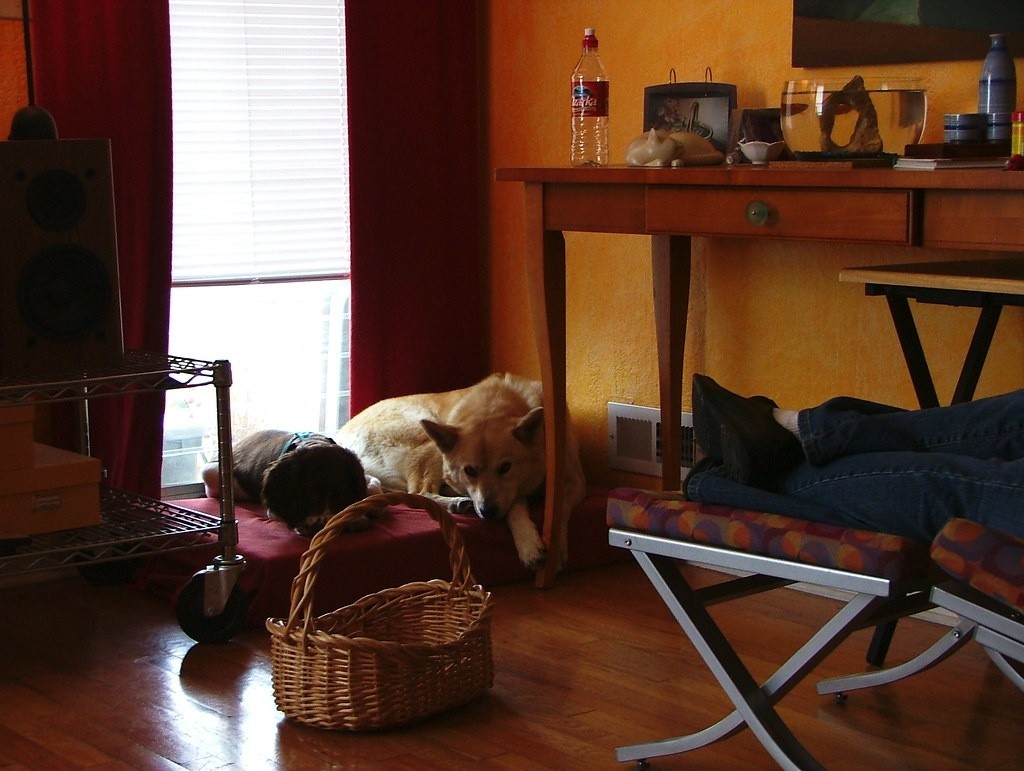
[204,430,369,539]
[338,370,587,569]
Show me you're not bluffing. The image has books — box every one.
[894,140,1009,171]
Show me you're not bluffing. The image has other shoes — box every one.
[691,373,806,486]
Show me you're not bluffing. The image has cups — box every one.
[943,113,988,142]
[987,113,1011,140]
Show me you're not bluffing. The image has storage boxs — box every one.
[779,77,928,161]
[0,379,101,537]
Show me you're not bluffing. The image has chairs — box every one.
[606,489,1024,771]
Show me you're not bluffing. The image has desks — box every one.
[495,157,1023,588]
[838,259,1024,666]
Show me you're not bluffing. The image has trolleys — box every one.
[0,138,252,648]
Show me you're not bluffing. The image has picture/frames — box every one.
[642,82,737,156]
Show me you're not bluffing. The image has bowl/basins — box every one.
[736,140,786,165]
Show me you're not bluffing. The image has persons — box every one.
[682,373,1024,538]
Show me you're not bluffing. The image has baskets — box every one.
[265,492,495,732]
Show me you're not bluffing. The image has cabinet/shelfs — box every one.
[1,347,261,645]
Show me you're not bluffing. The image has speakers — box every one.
[0,139,124,369]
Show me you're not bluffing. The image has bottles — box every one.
[1012,112,1024,157]
[569,28,612,167]
[977,33,1017,113]
